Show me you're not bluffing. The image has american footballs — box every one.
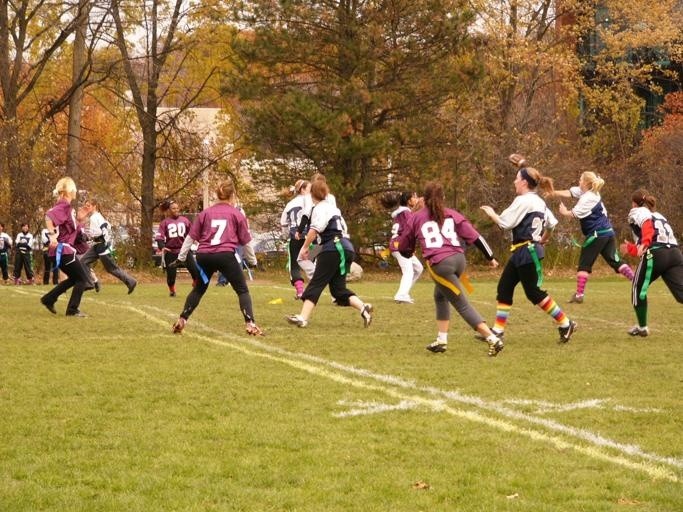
[508,153,528,168]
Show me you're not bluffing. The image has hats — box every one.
[294,179,304,191]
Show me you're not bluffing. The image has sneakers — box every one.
[626,327,648,337]
[94,281,101,293]
[557,319,577,344]
[28,279,33,284]
[332,299,337,305]
[284,314,309,329]
[474,328,504,342]
[14,279,19,285]
[170,292,176,296]
[172,318,186,334]
[360,303,373,328]
[127,282,137,294]
[66,312,86,316]
[41,299,57,314]
[5,280,10,285]
[426,342,448,355]
[567,293,584,304]
[294,294,304,302]
[488,339,504,357]
[246,324,265,337]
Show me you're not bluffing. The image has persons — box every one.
[380,188,424,304]
[168,183,265,336]
[619,189,683,337]
[80,199,137,295]
[543,171,634,303]
[218,204,245,288]
[398,182,504,357]
[155,201,200,296]
[40,177,88,316]
[0,221,14,284]
[74,224,99,292]
[42,228,59,286]
[312,174,336,209]
[285,181,373,328]
[475,167,577,344]
[13,223,35,285]
[280,180,312,299]
